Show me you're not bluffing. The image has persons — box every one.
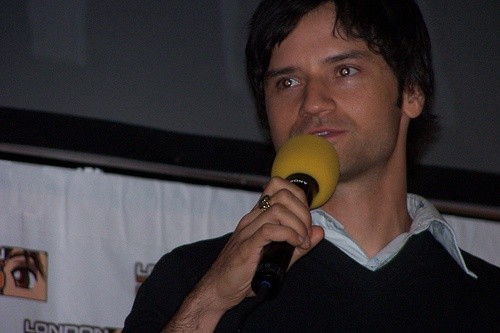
[119,0,500,333]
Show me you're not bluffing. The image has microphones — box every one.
[251,135,340,300]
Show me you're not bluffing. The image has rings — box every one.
[258,195,271,210]
